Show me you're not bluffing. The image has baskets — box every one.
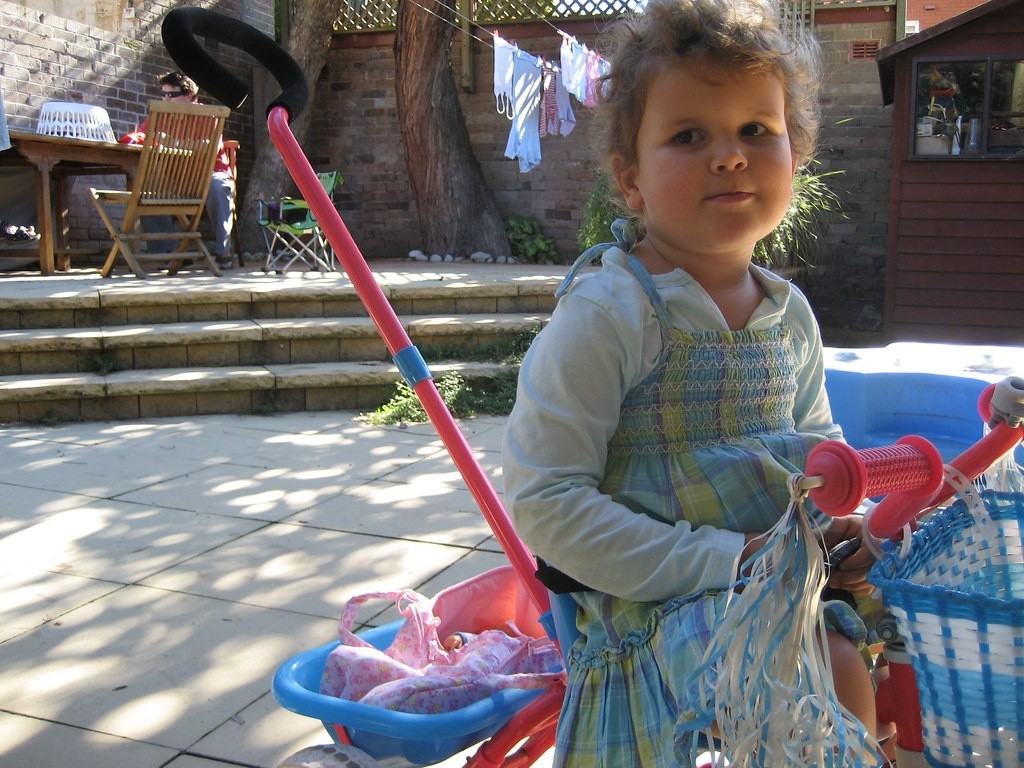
[867,489,1024,768]
[36,100,119,144]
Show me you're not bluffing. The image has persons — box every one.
[502,0,897,768]
[117,72,237,269]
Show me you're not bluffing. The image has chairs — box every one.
[258,172,339,276]
[88,100,240,279]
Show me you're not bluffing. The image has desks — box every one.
[7,131,194,275]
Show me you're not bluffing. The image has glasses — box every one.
[160,91,182,98]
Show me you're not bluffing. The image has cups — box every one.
[965,118,982,149]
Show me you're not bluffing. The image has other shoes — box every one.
[216,240,235,271]
